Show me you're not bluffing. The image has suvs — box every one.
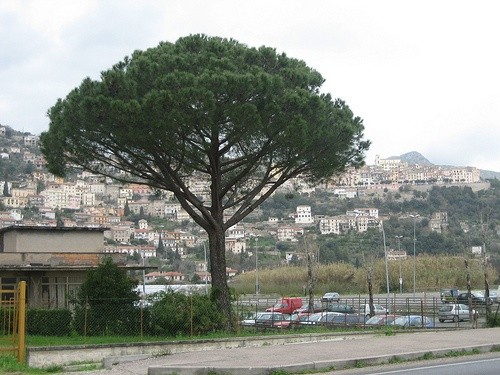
[438,303,479,323]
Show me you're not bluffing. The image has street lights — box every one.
[409,214,420,302]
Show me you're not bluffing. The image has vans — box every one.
[440,288,460,304]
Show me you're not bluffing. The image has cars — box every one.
[320,292,341,303]
[456,291,500,305]
[238,297,368,328]
[361,315,403,327]
[389,315,432,327]
[356,304,389,319]
